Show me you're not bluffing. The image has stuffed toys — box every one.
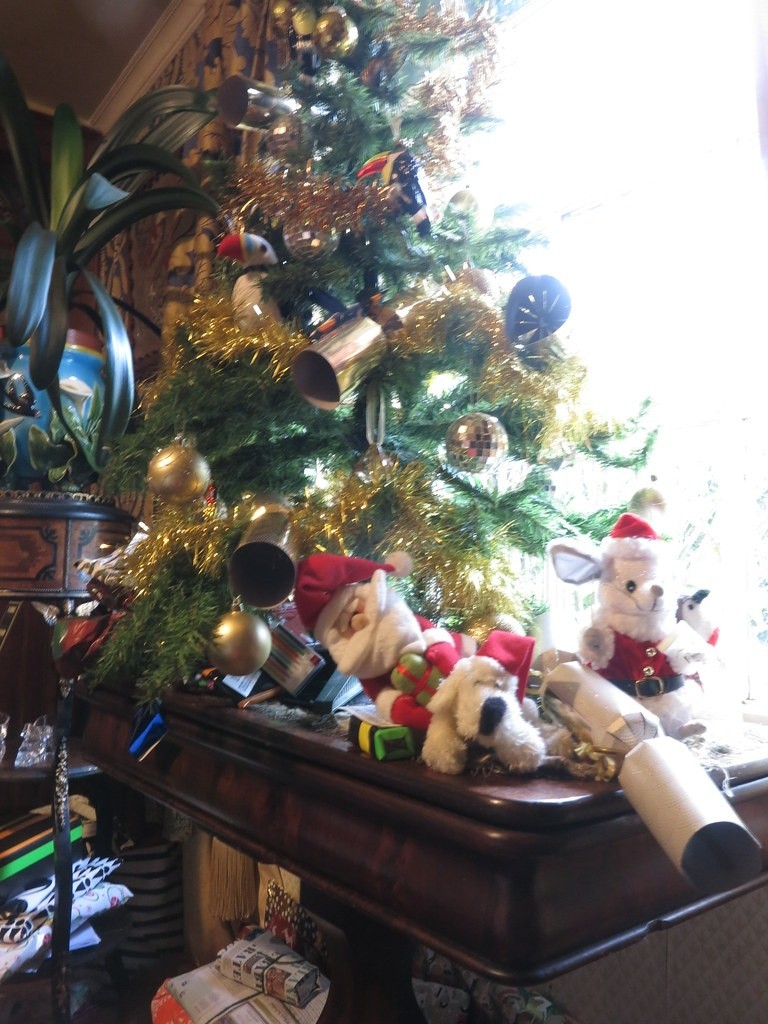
[420,655,576,776]
[219,232,348,334]
[293,552,536,734]
[546,513,722,741]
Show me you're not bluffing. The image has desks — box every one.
[70,686,768,1024]
